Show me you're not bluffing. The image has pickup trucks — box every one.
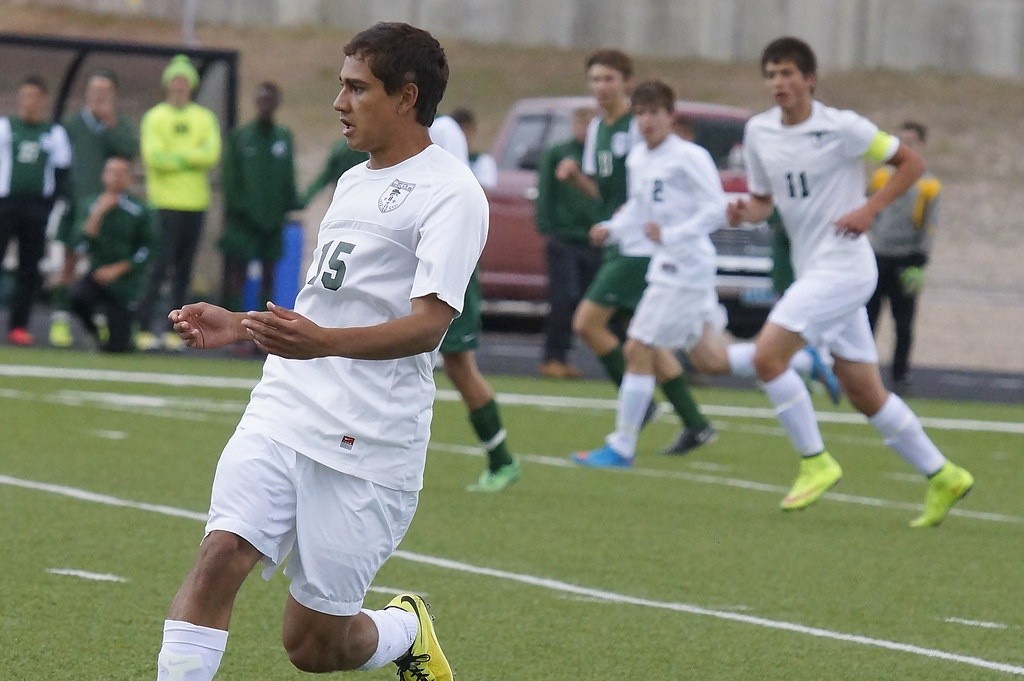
[465,93,777,320]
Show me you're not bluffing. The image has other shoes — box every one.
[8,328,34,345]
[539,357,577,377]
[163,328,184,352]
[135,332,160,351]
[49,320,72,347]
[98,323,110,343]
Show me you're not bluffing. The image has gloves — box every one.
[899,264,922,298]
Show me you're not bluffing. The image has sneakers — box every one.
[911,462,974,529]
[383,593,457,681]
[805,344,840,405]
[467,456,520,493]
[641,399,660,430]
[569,445,634,468]
[781,451,843,513]
[662,423,718,456]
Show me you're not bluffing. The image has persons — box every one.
[426,112,522,491]
[867,123,939,392]
[536,104,631,378]
[450,110,497,184]
[569,79,841,467]
[221,82,301,356]
[0,76,70,344]
[155,21,491,681]
[137,54,222,350]
[298,138,370,216]
[556,49,713,453]
[48,151,152,353]
[61,71,139,339]
[726,38,975,526]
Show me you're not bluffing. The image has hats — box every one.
[162,53,199,91]
